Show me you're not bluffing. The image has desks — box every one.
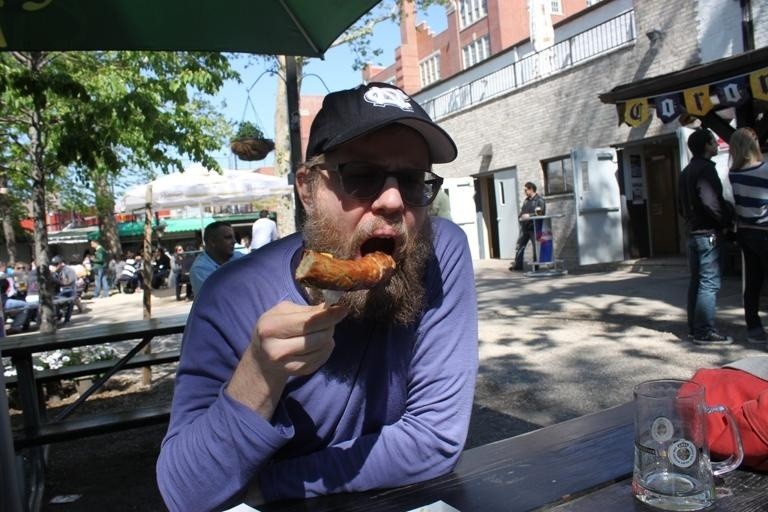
[247,368,766,512]
[518,213,567,277]
[0,313,190,512]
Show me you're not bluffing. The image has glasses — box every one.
[311,159,444,207]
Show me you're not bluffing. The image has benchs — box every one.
[11,403,171,456]
[6,350,182,425]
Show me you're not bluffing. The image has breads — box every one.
[294,249,397,291]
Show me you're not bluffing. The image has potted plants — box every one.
[228,119,273,162]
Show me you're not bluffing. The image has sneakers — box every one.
[692,329,733,345]
[745,328,767,344]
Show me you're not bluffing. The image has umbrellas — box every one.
[0,0,383,62]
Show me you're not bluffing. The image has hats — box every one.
[305,82,457,167]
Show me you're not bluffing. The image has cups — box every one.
[534,206,542,215]
[632,377,743,512]
[19,280,25,289]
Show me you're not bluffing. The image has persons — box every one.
[249,208,279,253]
[166,244,194,301]
[677,127,737,346]
[721,127,768,346]
[508,181,546,271]
[188,220,245,296]
[154,80,478,512]
[0,239,171,336]
